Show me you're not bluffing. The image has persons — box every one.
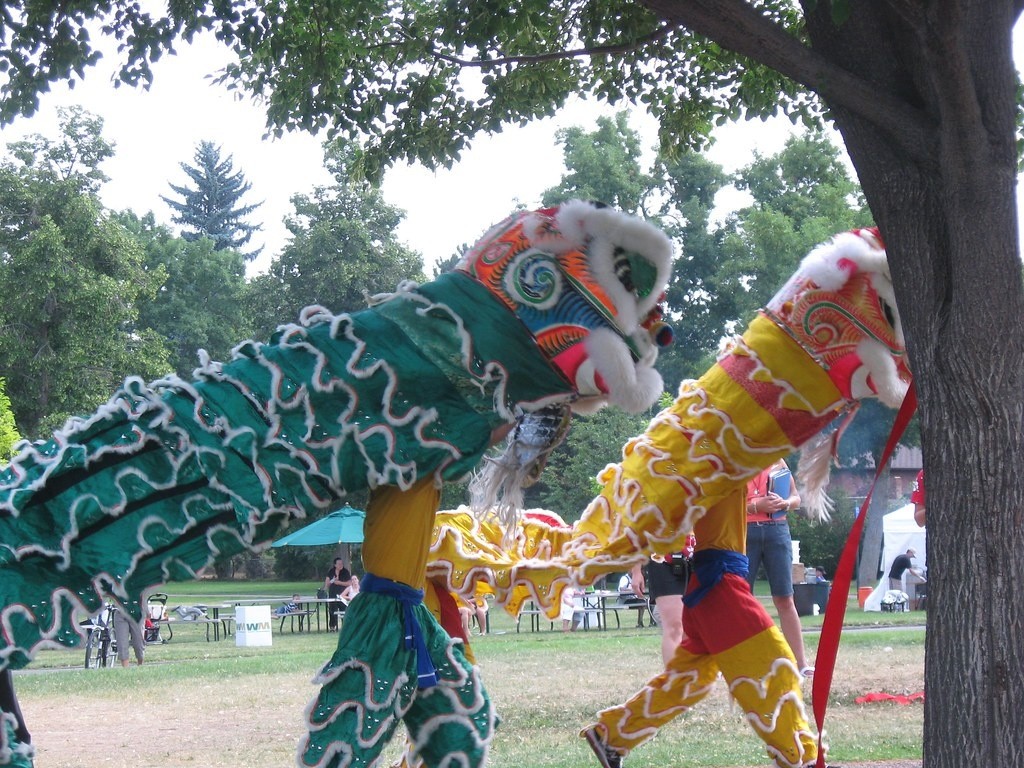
[889,547,926,591]
[325,557,352,632]
[113,594,144,669]
[561,586,585,632]
[816,566,832,589]
[448,590,487,638]
[295,418,520,768]
[914,469,926,527]
[145,611,157,629]
[745,460,818,677]
[580,460,840,768]
[271,594,300,620]
[340,575,361,605]
[392,576,478,768]
[616,525,696,715]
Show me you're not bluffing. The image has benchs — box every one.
[159,609,318,640]
[514,605,641,632]
[471,611,491,632]
[334,611,344,619]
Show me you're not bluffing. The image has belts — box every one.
[747,521,784,526]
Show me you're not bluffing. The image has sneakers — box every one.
[585,727,623,768]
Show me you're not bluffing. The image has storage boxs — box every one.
[790,540,828,617]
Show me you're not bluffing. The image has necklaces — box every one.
[751,474,761,495]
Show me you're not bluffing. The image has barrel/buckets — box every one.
[858,586,873,607]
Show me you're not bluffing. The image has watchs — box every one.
[783,500,790,512]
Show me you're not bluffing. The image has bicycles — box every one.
[82,602,121,669]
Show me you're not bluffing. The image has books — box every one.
[768,467,792,519]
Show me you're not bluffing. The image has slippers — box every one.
[800,666,815,677]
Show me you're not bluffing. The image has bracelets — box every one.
[751,498,757,514]
[746,508,751,514]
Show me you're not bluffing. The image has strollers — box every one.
[145,593,169,646]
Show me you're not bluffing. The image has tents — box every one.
[882,503,928,593]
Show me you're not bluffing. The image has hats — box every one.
[817,565,826,574]
[908,548,916,558]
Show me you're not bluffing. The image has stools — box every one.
[880,602,905,611]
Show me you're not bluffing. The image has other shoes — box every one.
[903,609,910,612]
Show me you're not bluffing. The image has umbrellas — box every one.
[271,501,369,580]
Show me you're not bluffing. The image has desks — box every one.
[571,593,622,631]
[293,597,342,633]
[225,597,317,634]
[914,583,928,610]
[164,604,232,641]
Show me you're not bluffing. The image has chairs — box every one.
[145,593,173,643]
